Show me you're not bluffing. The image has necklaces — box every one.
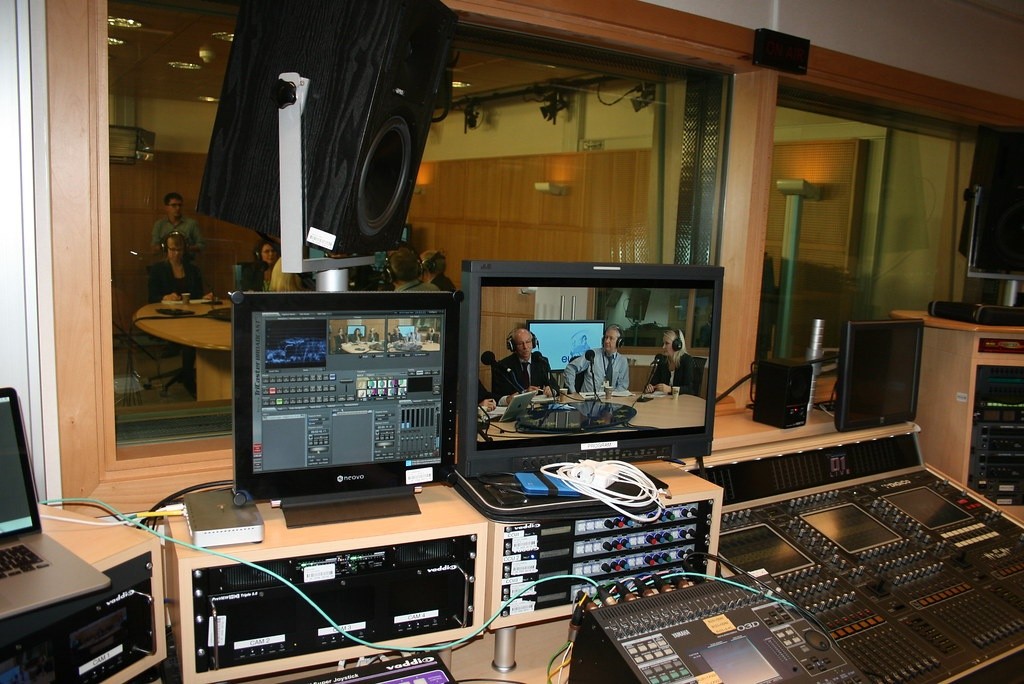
[671,365,676,370]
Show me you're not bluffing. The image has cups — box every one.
[560,389,568,401]
[605,387,613,400]
[182,293,190,304]
[672,387,680,400]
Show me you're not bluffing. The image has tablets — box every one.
[157,308,195,315]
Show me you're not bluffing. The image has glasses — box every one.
[169,203,182,207]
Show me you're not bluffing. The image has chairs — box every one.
[693,355,708,396]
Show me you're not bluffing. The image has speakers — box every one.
[958,124,1024,271]
[195,0,458,255]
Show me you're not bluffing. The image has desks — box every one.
[132,299,231,401]
[478,389,706,444]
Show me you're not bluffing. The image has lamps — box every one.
[631,82,656,112]
[540,93,565,118]
[462,101,479,129]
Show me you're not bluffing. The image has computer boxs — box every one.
[753,357,813,429]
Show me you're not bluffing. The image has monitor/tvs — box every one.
[833,317,924,432]
[458,260,724,476]
[347,325,366,338]
[397,325,416,338]
[525,320,606,373]
[230,288,465,529]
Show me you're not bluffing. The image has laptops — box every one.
[478,390,536,422]
[0,388,111,619]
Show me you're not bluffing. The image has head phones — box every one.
[506,327,538,352]
[421,251,446,273]
[672,330,682,351]
[253,249,262,262]
[160,230,192,252]
[602,324,625,348]
[381,237,423,283]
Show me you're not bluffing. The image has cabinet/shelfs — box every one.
[892,310,1024,521]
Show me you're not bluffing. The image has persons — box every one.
[241,239,457,292]
[645,329,695,395]
[477,376,519,412]
[492,328,560,406]
[390,325,439,343]
[147,193,216,358]
[562,324,629,394]
[336,328,379,354]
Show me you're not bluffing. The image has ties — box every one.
[605,355,613,386]
[520,361,530,387]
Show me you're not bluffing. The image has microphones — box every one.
[585,349,596,364]
[481,351,512,373]
[650,353,663,365]
